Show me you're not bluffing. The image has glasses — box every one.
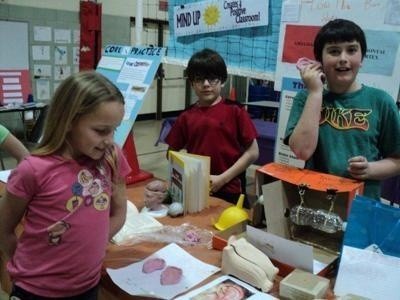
[192,76,219,86]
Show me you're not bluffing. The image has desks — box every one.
[0,103,49,142]
[0,169,335,300]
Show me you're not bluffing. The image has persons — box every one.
[0,71,134,300]
[283,19,400,202]
[0,123,30,164]
[143,180,167,211]
[164,49,260,209]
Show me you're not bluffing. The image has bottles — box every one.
[289,205,348,233]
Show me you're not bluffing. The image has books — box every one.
[168,150,212,216]
[110,198,163,246]
